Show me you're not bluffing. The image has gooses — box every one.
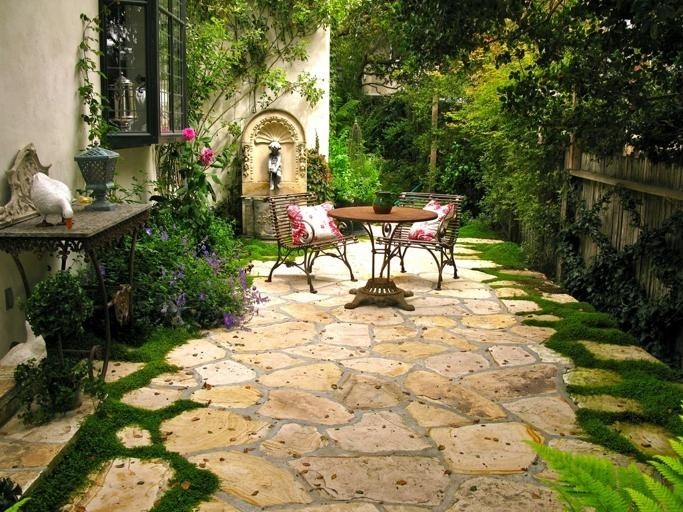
[30,172,74,230]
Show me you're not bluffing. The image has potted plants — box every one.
[22,274,95,408]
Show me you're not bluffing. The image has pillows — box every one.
[408,201,455,241]
[285,201,346,245]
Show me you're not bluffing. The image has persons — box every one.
[266,142,283,192]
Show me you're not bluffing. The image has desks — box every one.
[0,203,153,385]
[328,207,437,311]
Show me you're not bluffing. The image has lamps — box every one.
[72,140,120,212]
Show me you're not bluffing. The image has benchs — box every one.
[378,192,465,290]
[265,192,357,292]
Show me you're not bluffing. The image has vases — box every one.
[371,192,393,213]
